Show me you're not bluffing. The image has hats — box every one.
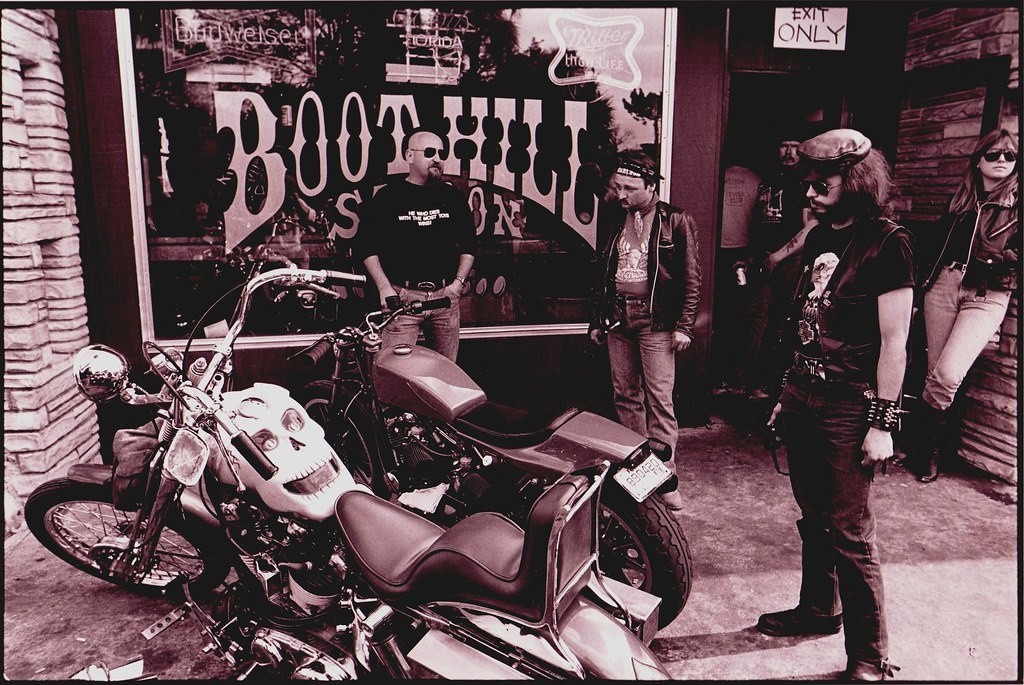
[796,128,872,171]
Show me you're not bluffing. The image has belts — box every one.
[389,274,457,292]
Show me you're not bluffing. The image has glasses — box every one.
[983,150,1017,162]
[795,177,840,197]
[410,147,447,161]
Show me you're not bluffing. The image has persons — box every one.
[586,150,701,509]
[712,130,807,398]
[758,128,922,682]
[908,128,1020,483]
[355,131,477,362]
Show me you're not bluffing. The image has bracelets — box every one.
[456,275,468,285]
[867,397,906,432]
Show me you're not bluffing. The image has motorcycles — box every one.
[23,212,674,682]
[287,295,695,631]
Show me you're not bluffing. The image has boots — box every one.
[900,393,950,482]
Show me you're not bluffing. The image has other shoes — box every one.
[757,605,842,636]
[838,658,900,685]
[745,388,769,400]
[709,383,745,398]
[660,489,684,510]
